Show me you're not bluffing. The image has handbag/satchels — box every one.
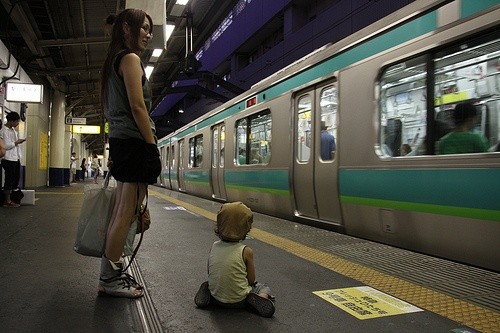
[72,169,142,259]
[138,202,150,234]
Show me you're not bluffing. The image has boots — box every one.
[97,254,144,297]
[128,275,144,289]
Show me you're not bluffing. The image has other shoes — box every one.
[246,291,276,318]
[4,202,21,207]
[193,281,212,308]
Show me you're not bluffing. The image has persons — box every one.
[81,158,86,182]
[93,154,100,185]
[70,152,79,183]
[0,112,24,209]
[194,201,275,317]
[239,146,263,165]
[97,8,162,298]
[320,121,336,161]
[393,144,412,157]
[193,141,203,168]
[438,102,489,154]
[220,146,224,166]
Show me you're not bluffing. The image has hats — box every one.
[214,201,254,242]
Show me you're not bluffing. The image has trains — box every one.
[147,0,500,274]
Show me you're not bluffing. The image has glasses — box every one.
[140,26,154,40]
